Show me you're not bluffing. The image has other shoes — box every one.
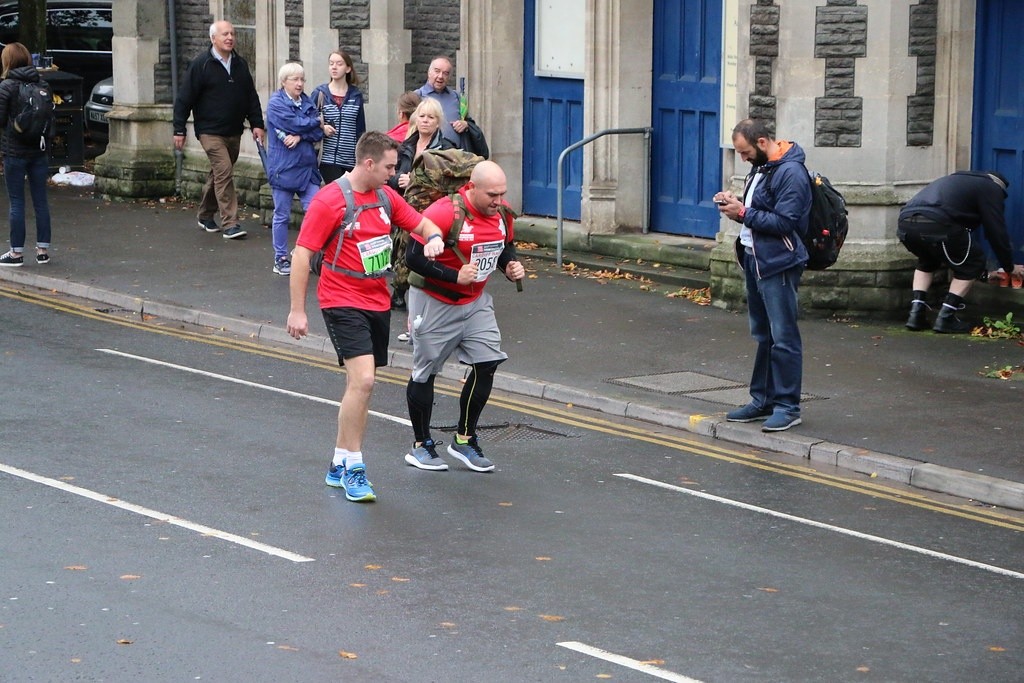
[397,331,411,341]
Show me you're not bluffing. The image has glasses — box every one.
[286,77,307,84]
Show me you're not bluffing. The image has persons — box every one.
[897,170,1024,333]
[286,131,446,503]
[383,98,459,311]
[712,118,814,431]
[381,91,422,141]
[0,43,52,267]
[410,57,489,160]
[310,50,366,187]
[171,20,264,239]
[265,62,324,275]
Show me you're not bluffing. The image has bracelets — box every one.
[737,208,746,222]
[427,234,442,240]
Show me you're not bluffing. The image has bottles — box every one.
[273,128,297,149]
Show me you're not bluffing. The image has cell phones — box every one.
[715,201,727,206]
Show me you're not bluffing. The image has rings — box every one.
[433,248,439,251]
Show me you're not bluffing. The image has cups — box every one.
[31,54,40,69]
[997,268,1009,287]
[1010,272,1022,289]
[43,57,53,67]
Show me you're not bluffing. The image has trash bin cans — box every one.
[39,70,85,172]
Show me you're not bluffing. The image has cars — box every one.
[83,76,116,139]
[0,0,113,75]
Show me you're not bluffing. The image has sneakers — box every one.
[36,253,51,264]
[405,439,448,470]
[197,219,220,232]
[223,227,247,239]
[0,252,24,266]
[340,463,376,501]
[761,413,802,431]
[726,403,773,422]
[447,434,494,471]
[932,313,970,334]
[905,311,931,331]
[272,256,291,275]
[325,458,373,487]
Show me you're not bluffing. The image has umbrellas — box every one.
[458,78,468,119]
[256,138,267,172]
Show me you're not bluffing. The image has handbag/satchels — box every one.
[312,91,324,169]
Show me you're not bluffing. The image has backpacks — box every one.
[744,161,848,271]
[309,178,392,276]
[5,78,52,152]
[390,149,509,290]
